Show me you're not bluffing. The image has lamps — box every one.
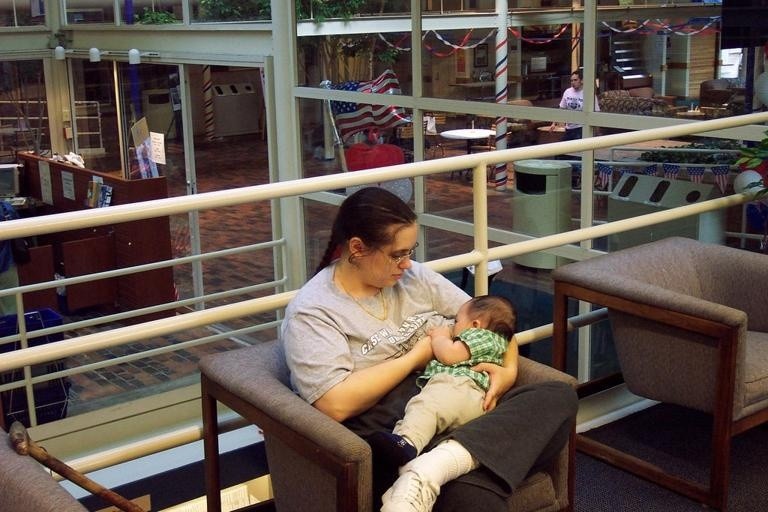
[52,45,160,67]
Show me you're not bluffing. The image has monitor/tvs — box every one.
[0,164,19,198]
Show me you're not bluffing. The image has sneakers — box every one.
[369,429,416,463]
[380,469,442,512]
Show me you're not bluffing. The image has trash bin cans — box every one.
[607,173,726,255]
[511,158,573,269]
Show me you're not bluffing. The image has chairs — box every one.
[0,427,81,511]
[388,121,427,151]
[194,327,567,511]
[548,232,762,510]
[469,130,512,178]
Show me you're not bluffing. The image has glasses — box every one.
[378,242,419,262]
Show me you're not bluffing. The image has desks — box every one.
[439,128,493,180]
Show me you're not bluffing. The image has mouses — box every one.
[27,199,35,207]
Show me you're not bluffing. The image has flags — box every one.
[325,70,412,143]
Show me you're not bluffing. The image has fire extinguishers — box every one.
[666,37,672,59]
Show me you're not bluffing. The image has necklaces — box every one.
[337,264,387,322]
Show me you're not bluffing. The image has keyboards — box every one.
[4,197,26,205]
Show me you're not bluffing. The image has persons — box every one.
[365,294,515,469]
[279,187,579,511]
[546,70,600,183]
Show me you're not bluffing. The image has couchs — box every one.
[501,79,744,138]
